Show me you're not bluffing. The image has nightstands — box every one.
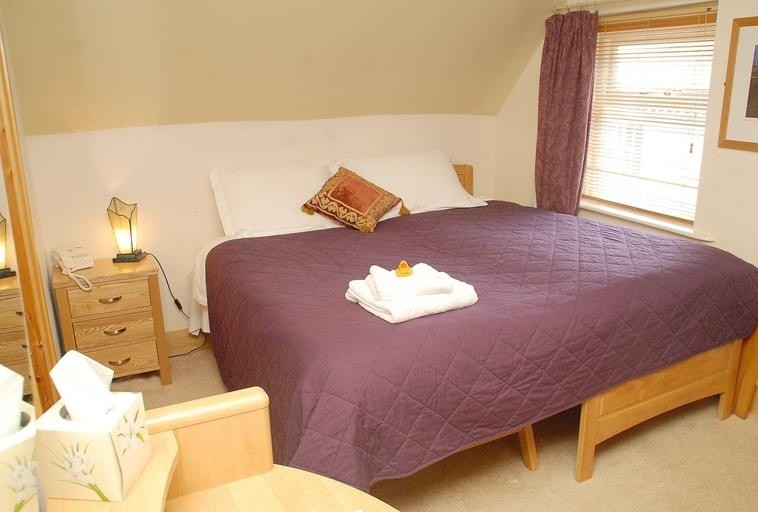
[51,255,172,385]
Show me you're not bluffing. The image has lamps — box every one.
[106,197,149,262]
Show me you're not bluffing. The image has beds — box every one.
[200,164,757,497]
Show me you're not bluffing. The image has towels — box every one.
[344,262,479,324]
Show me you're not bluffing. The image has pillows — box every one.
[208,149,490,238]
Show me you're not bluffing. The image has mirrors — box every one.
[1,54,57,511]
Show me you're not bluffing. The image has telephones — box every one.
[53,245,95,272]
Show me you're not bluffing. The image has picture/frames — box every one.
[716,16,758,153]
